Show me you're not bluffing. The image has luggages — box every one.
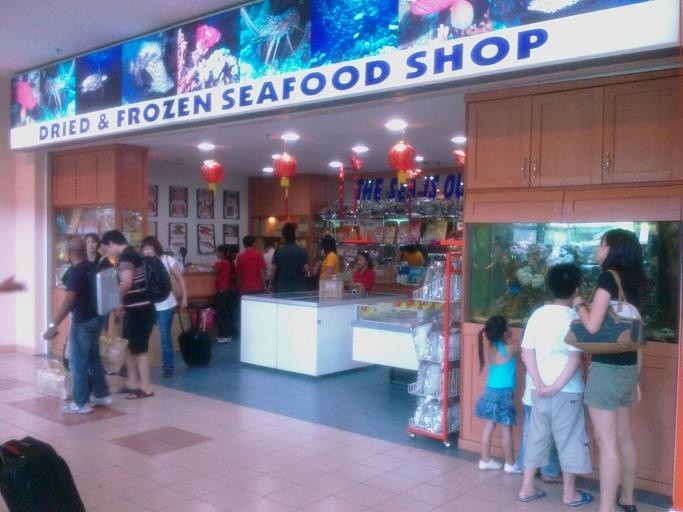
[1,431,89,512]
[177,327,213,369]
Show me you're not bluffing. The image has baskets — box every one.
[64,328,130,375]
[37,359,74,399]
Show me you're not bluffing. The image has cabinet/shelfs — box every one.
[246,175,334,251]
[48,142,151,368]
[320,202,463,292]
[605,74,682,187]
[456,320,678,497]
[406,237,464,448]
[463,83,605,190]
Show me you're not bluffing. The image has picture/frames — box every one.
[147,182,242,257]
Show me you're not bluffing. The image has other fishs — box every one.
[240,1,305,68]
[41,60,74,112]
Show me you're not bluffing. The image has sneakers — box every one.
[477,457,503,471]
[216,335,234,345]
[503,462,522,474]
[60,393,115,414]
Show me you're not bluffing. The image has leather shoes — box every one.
[615,495,637,512]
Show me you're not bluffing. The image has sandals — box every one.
[567,488,595,507]
[516,485,546,503]
[114,383,155,399]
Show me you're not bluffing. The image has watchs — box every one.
[574,303,585,313]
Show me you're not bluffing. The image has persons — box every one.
[476,316,522,472]
[41,230,191,414]
[212,222,374,344]
[572,230,648,512]
[516,264,594,506]
[513,369,565,484]
[401,245,426,268]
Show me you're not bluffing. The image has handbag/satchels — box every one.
[270,273,281,294]
[562,266,648,357]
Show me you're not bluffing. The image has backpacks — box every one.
[87,256,121,318]
[140,255,171,304]
